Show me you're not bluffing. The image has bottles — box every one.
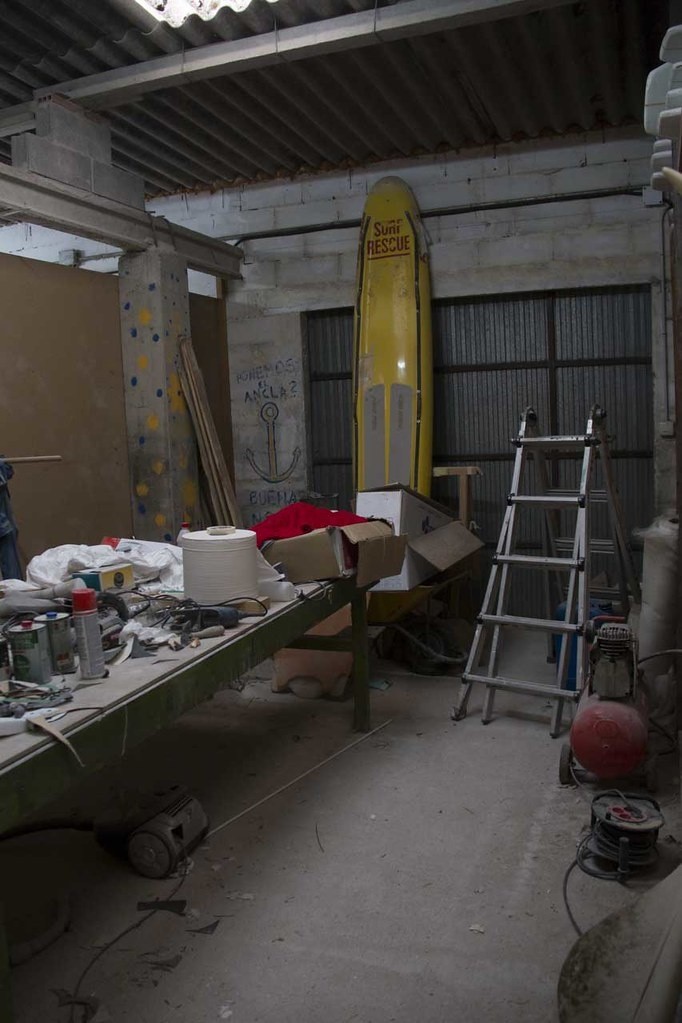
[72,588,106,679]
[177,522,191,548]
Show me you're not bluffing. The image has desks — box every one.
[1,569,381,839]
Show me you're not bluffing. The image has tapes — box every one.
[206,525,236,535]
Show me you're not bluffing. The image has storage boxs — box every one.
[259,481,485,691]
[72,563,134,593]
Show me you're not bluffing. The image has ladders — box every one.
[448,401,643,738]
